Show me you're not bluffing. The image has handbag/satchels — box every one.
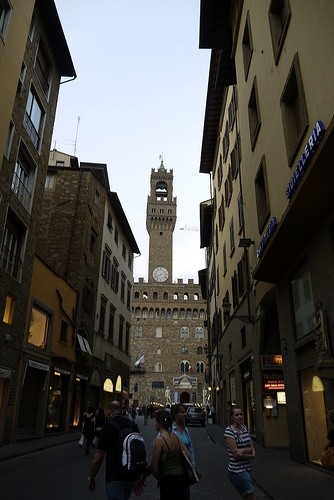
[182,451,199,485]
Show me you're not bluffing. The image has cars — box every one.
[184,407,206,427]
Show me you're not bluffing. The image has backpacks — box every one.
[110,420,147,475]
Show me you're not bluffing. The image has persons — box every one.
[156,414,196,471]
[133,410,192,500]
[223,405,257,500]
[79,401,216,449]
[88,401,146,500]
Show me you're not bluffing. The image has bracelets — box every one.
[88,476,96,480]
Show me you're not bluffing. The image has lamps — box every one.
[280,338,287,350]
[216,386,223,394]
[208,385,217,392]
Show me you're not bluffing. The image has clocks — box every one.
[152,266,169,283]
